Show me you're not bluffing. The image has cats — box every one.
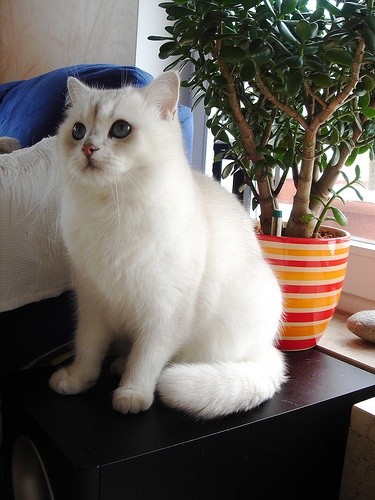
[47,68,288,418]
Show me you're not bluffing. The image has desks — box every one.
[0,134,375,500]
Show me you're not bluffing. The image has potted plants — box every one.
[147,0,375,352]
